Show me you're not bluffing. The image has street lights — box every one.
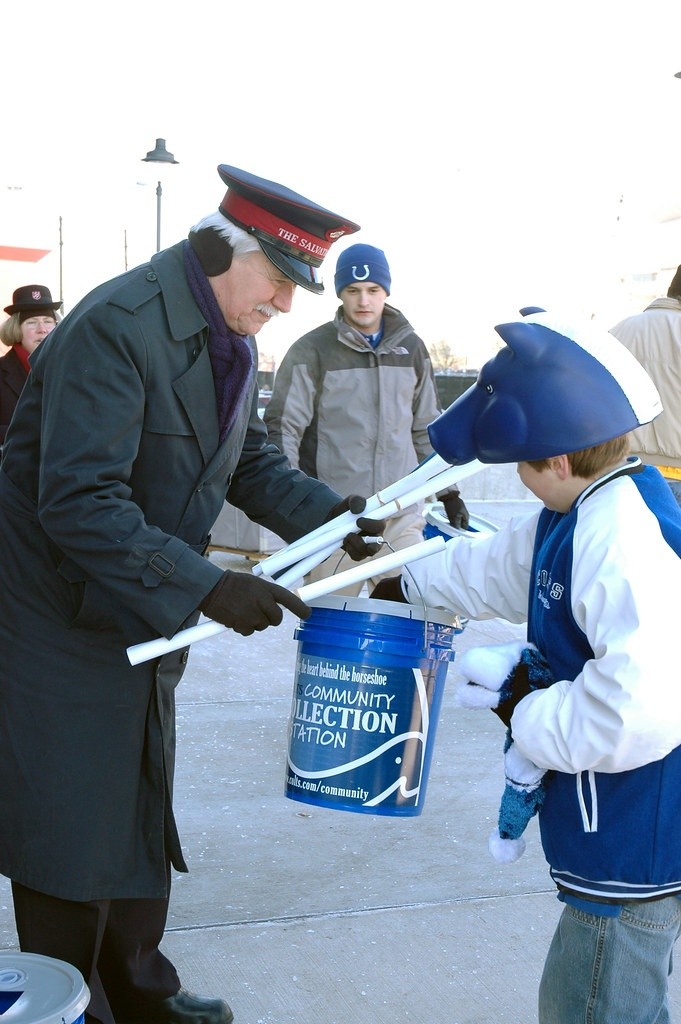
[143,136,180,255]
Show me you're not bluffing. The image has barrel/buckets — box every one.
[285,537,461,817]
[422,505,503,637]
[0,950,91,1024]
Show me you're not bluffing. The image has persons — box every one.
[369,305,681,1024]
[0,284,64,452]
[0,164,387,1024]
[608,265,681,505]
[264,242,470,598]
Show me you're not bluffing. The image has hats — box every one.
[334,243,392,296]
[217,164,361,295]
[426,307,664,466]
[4,285,63,317]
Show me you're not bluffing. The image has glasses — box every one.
[25,317,58,329]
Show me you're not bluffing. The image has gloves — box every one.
[326,494,385,562]
[443,497,469,531]
[369,574,409,605]
[490,663,531,728]
[198,569,311,637]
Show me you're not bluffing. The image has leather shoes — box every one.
[116,990,234,1024]
